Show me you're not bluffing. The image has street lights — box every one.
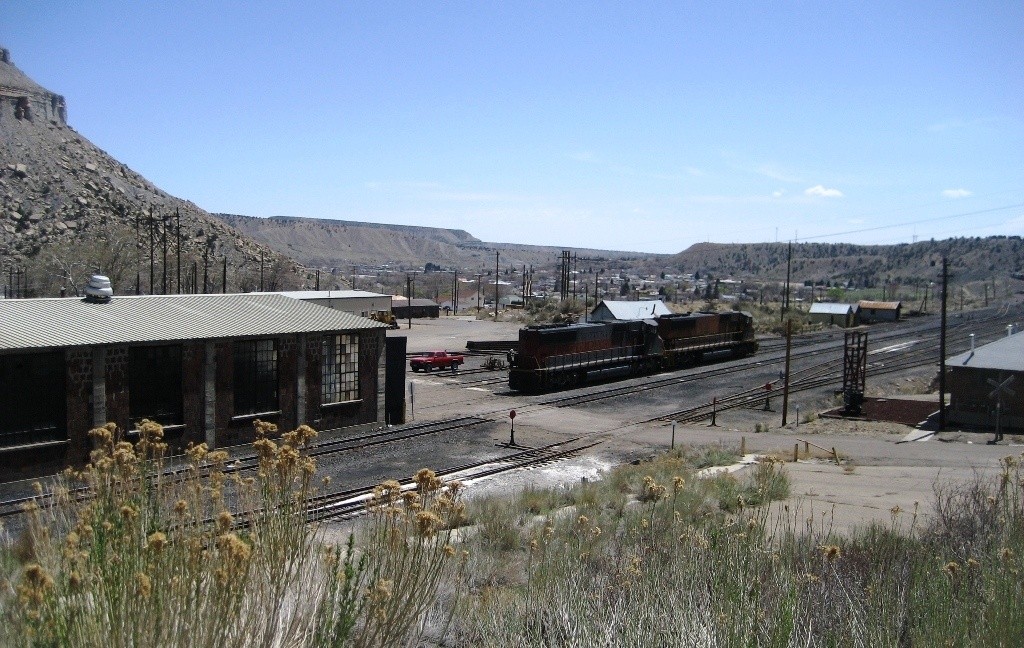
[928,254,948,430]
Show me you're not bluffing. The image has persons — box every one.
[507,348,515,366]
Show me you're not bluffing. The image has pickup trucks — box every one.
[410,350,466,373]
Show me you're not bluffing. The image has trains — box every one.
[503,309,760,393]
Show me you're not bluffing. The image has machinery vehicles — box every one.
[369,309,401,330]
